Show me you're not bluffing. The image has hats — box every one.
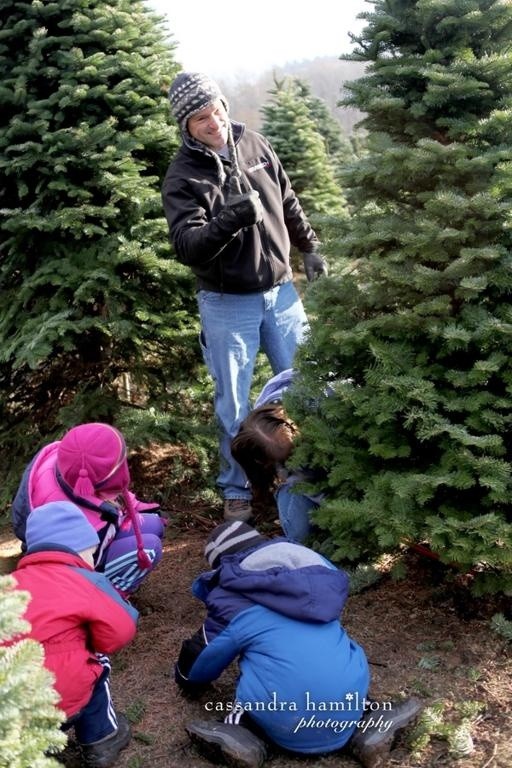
[58,422,131,498]
[203,520,265,570]
[169,72,229,139]
[25,500,101,552]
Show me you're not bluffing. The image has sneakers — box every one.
[349,696,424,768]
[78,721,132,768]
[223,498,252,522]
[183,717,268,768]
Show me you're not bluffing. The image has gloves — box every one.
[227,176,264,229]
[302,249,329,282]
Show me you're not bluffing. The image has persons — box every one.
[174,519,423,767]
[2,500,141,767]
[229,362,356,546]
[10,422,169,615]
[161,71,331,521]
